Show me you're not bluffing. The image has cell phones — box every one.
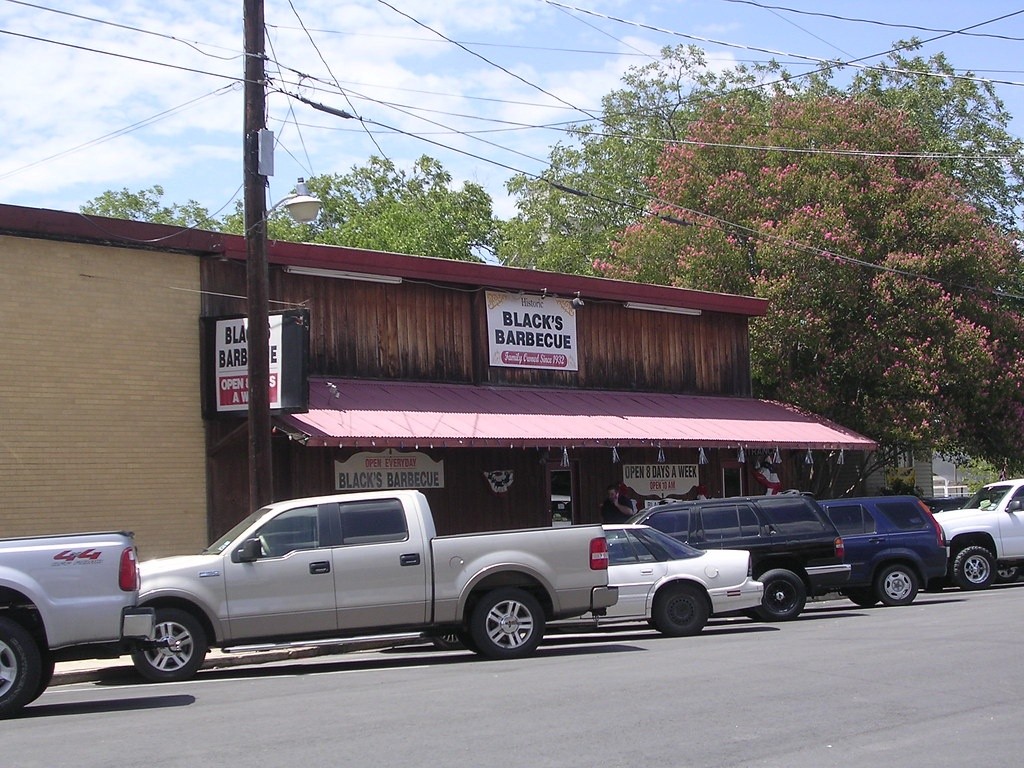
[616,493,620,498]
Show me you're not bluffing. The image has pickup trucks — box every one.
[0,530,173,721]
[133,488,619,684]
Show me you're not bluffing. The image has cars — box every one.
[430,524,766,650]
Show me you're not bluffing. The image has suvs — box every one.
[607,489,852,623]
[815,494,949,608]
[929,479,1024,592]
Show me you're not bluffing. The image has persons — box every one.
[602,486,633,523]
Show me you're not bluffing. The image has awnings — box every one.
[208,376,879,457]
